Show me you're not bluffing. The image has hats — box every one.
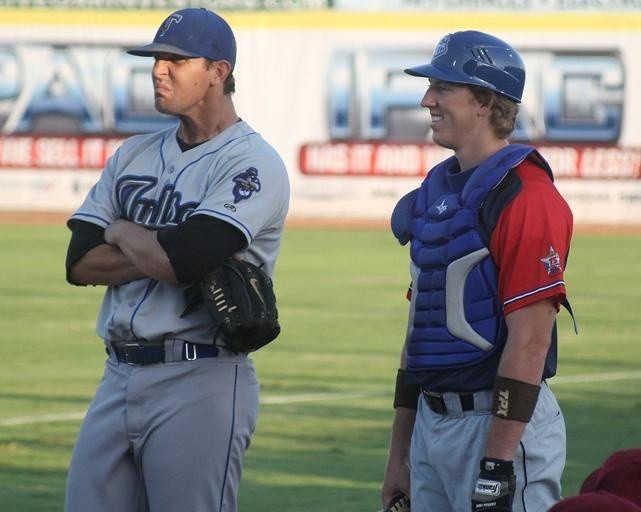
[127,8,235,72]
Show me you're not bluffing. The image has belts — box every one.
[421,385,473,414]
[105,341,219,366]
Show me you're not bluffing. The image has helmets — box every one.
[404,31,525,104]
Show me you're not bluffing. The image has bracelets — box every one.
[394,369,418,409]
[491,376,541,423]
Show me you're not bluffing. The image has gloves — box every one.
[472,456,515,512]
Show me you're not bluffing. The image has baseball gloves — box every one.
[202,256,281,357]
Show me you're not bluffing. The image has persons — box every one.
[65,7,290,512]
[383,30,573,512]
